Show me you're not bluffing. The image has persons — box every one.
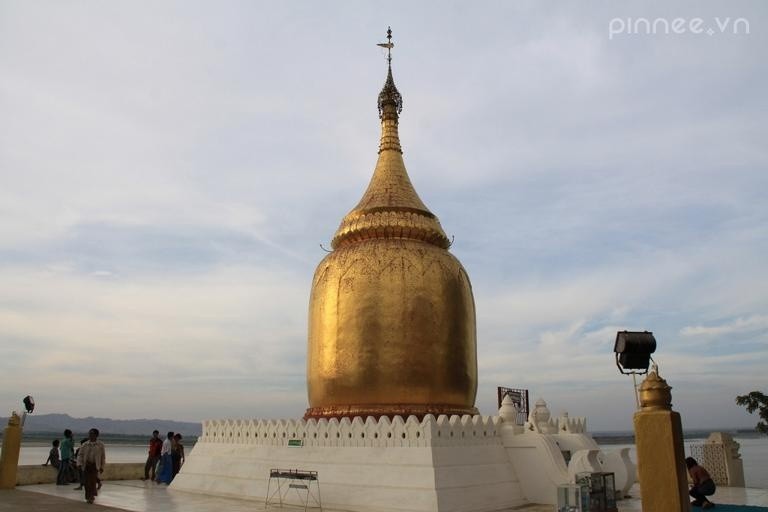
[141,429,185,484]
[684,456,716,510]
[40,427,106,504]
